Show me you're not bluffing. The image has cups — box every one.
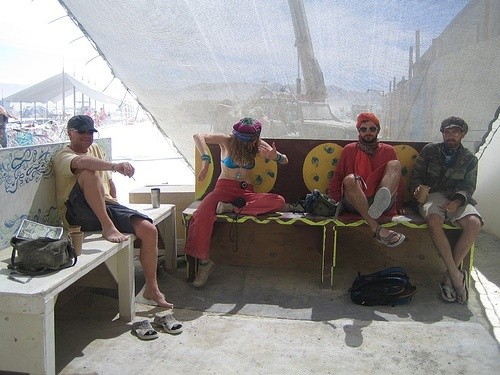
[417,184,431,203]
[151,188,160,208]
[68,225,81,232]
[68,231,84,256]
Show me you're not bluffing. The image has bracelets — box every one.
[201,154,212,165]
[274,154,285,164]
[111,163,116,173]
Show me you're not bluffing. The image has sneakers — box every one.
[192,259,216,288]
[215,201,234,215]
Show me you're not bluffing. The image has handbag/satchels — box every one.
[347,265,418,308]
[7,234,77,285]
[304,189,344,217]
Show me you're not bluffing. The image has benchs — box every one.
[182,138,475,288]
[0,138,177,375]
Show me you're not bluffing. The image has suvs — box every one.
[259,95,356,139]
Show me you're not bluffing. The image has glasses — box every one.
[359,127,376,132]
[73,129,94,134]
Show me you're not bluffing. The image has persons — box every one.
[53,115,174,309]
[185,117,289,288]
[328,112,406,249]
[0,106,18,149]
[406,116,484,304]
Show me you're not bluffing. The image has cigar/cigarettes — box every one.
[131,176,135,181]
[447,209,450,213]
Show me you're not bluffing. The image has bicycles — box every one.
[6,120,70,147]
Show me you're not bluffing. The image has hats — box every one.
[67,114,98,133]
[356,112,380,129]
[232,117,262,142]
[440,116,469,136]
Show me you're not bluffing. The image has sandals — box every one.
[373,225,406,248]
[367,186,392,220]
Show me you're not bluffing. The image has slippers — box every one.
[454,269,470,304]
[438,280,457,303]
[131,319,159,341]
[154,312,184,335]
